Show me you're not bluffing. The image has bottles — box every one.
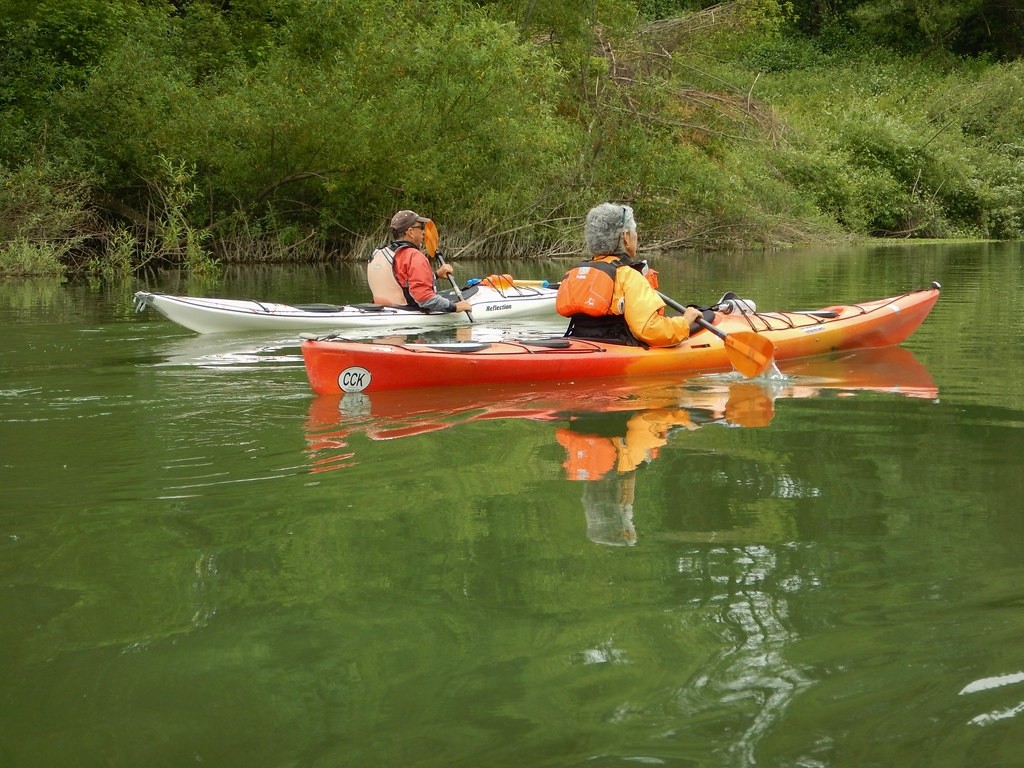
[479,274,513,287]
[718,299,757,317]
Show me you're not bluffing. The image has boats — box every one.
[136,269,664,335]
[297,282,943,396]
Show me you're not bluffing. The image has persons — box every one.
[366,210,472,312]
[556,202,704,348]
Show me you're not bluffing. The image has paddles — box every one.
[646,283,791,383]
[416,212,484,327]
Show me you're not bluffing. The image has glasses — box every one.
[412,224,425,230]
[621,206,628,228]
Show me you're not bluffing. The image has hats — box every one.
[391,210,431,232]
